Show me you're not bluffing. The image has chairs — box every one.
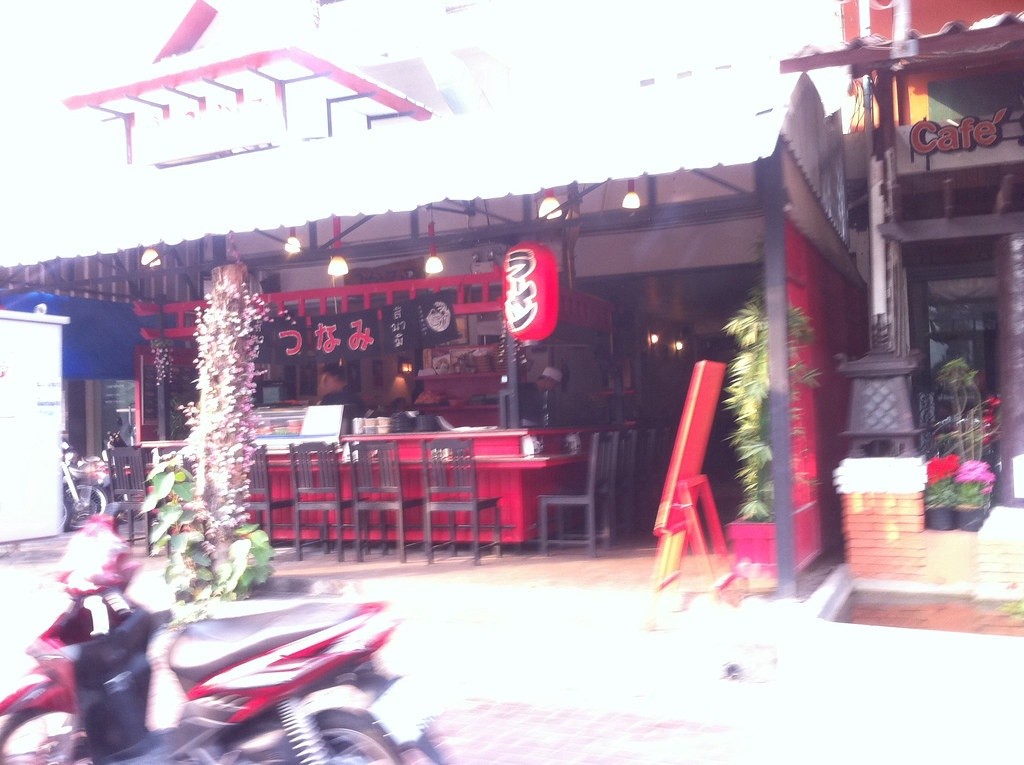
[534,430,620,559]
[106,447,164,556]
[657,427,675,466]
[636,428,656,535]
[348,439,425,564]
[159,444,219,559]
[420,436,502,566]
[286,440,371,563]
[596,428,638,546]
[233,443,294,562]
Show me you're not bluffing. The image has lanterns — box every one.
[502,240,559,344]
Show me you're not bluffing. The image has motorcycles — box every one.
[1,494,458,764]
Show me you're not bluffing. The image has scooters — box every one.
[56,399,143,535]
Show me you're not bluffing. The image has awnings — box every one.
[1,66,798,267]
[779,20,1024,84]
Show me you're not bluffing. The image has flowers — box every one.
[927,454,959,500]
[980,397,1002,446]
[953,460,996,507]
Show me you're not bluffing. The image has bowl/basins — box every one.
[377,417,391,425]
[377,425,391,434]
[365,425,377,435]
[363,418,377,426]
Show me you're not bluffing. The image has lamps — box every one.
[140,246,162,267]
[283,227,301,254]
[538,188,563,220]
[424,222,445,274]
[621,180,641,209]
[326,216,349,277]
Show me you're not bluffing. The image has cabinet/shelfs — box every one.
[413,371,505,410]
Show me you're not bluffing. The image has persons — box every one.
[518,366,563,429]
[315,366,359,419]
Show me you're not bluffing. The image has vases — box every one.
[956,506,986,533]
[927,508,956,531]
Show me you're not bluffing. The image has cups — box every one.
[353,417,365,433]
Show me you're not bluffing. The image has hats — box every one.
[541,366,563,382]
